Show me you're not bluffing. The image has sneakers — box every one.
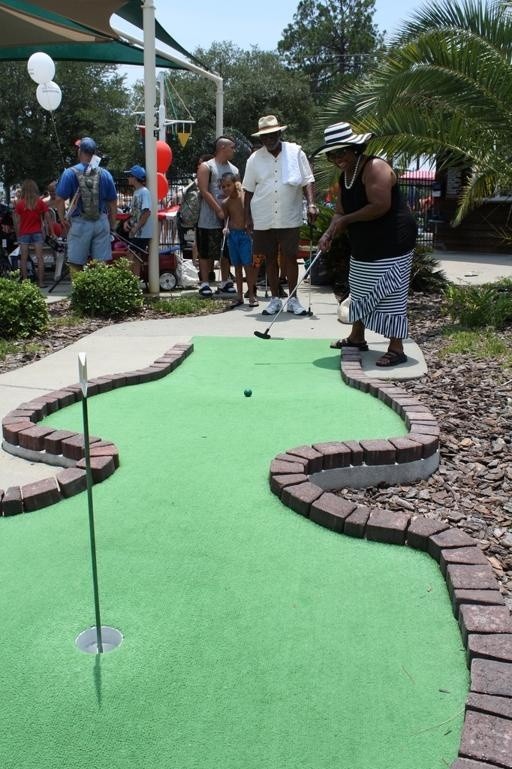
[245,286,259,298]
[262,297,282,315]
[286,296,306,315]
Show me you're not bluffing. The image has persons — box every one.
[243,114,321,316]
[120,164,154,287]
[196,135,240,297]
[218,172,259,308]
[1,203,13,267]
[53,137,119,287]
[45,180,65,218]
[10,178,58,290]
[307,119,420,368]
[192,154,216,283]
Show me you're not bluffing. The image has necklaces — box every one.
[340,154,363,190]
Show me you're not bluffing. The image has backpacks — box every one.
[73,170,100,222]
[177,177,200,228]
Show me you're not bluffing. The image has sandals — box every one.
[374,350,407,366]
[330,337,369,351]
[231,299,244,307]
[217,279,237,293]
[248,296,259,307]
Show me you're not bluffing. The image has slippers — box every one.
[199,284,212,296]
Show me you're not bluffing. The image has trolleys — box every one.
[110,229,179,293]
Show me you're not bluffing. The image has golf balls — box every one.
[244,388,252,399]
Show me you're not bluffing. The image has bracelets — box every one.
[306,203,317,209]
[323,232,332,239]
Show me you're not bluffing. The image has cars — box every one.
[117,193,133,208]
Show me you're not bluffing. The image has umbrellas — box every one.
[373,149,437,211]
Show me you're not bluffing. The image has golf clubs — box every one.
[254,251,321,339]
[215,214,230,292]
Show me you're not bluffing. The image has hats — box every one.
[75,137,96,152]
[251,115,288,137]
[123,164,145,180]
[310,121,374,159]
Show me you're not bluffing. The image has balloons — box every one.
[47,222,62,236]
[26,50,56,86]
[35,81,63,113]
[156,172,168,201]
[156,140,174,174]
